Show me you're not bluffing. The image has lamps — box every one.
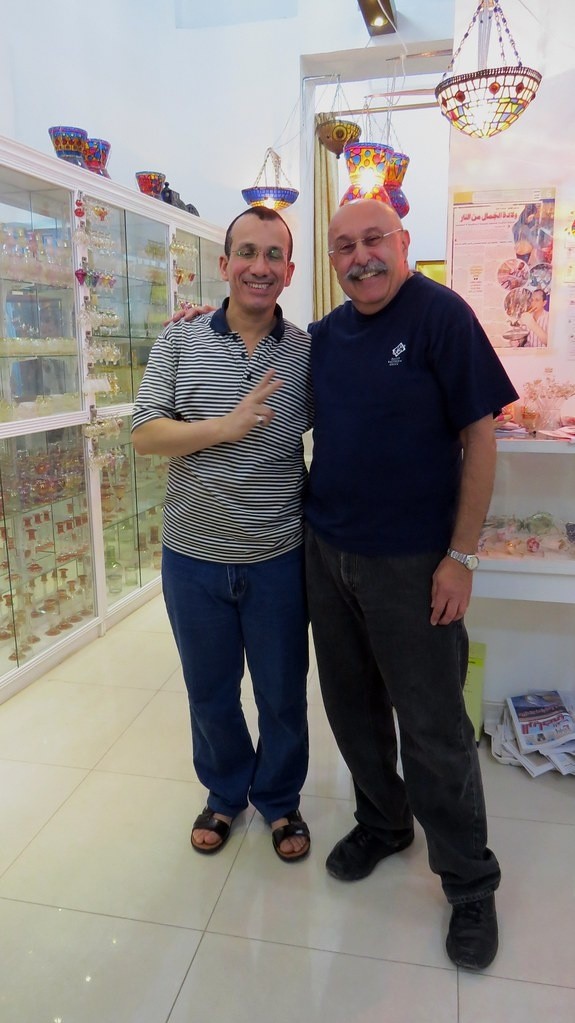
[380,96,410,219]
[339,95,394,207]
[241,147,300,211]
[434,0,542,139]
[316,76,362,159]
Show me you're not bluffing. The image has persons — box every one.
[128,205,317,862]
[164,195,521,971]
[510,289,549,348]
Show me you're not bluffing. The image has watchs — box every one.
[445,547,480,571]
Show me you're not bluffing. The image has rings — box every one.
[256,414,265,423]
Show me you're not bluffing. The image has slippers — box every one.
[271,808,311,861]
[191,803,233,853]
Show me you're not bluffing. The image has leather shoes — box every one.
[447,891,498,969]
[326,820,417,884]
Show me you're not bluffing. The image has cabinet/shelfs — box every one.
[470,439,575,603]
[0,135,229,704]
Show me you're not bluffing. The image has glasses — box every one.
[228,245,290,266]
[327,227,408,255]
